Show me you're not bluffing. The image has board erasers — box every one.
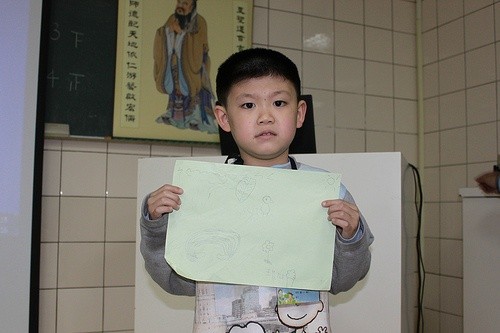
[42,120,71,139]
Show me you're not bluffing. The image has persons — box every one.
[138,45,375,333]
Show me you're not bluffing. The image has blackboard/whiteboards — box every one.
[42,0,119,142]
